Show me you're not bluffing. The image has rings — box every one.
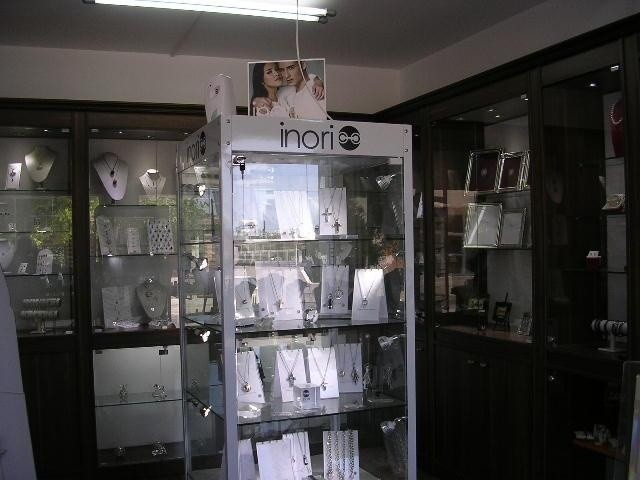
[37,253,49,273]
[149,220,173,251]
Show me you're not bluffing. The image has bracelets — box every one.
[593,319,627,337]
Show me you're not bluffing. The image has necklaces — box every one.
[276,191,306,239]
[279,351,300,387]
[145,173,162,190]
[235,266,248,305]
[103,155,119,184]
[324,265,344,309]
[356,269,376,306]
[610,99,623,126]
[268,267,287,310]
[33,149,45,170]
[310,348,331,391]
[328,431,355,479]
[285,431,308,465]
[337,343,360,386]
[236,351,254,393]
[388,423,408,472]
[320,188,343,232]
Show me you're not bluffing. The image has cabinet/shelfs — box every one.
[376,15,638,479]
[172,116,419,478]
[0,96,188,480]
